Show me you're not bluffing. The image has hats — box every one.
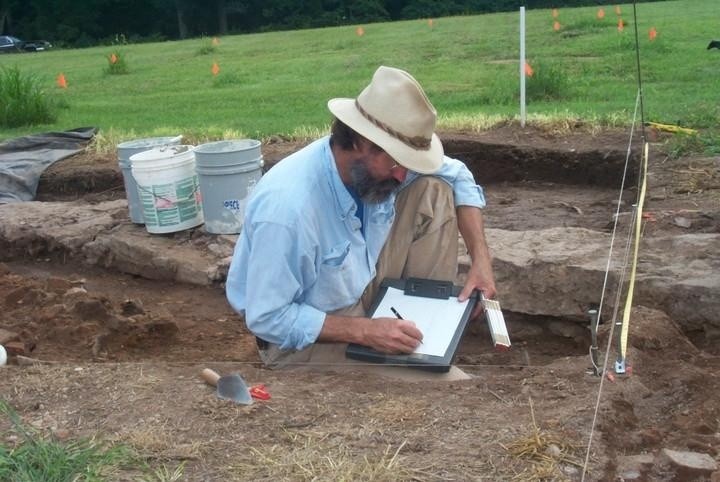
[328,65,445,175]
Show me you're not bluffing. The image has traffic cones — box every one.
[110,53,118,64]
[213,37,220,45]
[357,26,364,36]
[211,62,219,74]
[57,71,66,87]
[525,5,658,78]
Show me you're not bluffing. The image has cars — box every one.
[0,36,52,54]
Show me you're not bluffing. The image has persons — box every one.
[225,65,498,383]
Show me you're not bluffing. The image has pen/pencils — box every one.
[391,307,424,345]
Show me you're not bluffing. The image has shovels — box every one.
[201,368,253,404]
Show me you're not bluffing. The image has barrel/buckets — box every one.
[130,146,204,234]
[195,139,265,235]
[116,136,184,225]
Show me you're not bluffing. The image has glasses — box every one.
[384,153,408,171]
